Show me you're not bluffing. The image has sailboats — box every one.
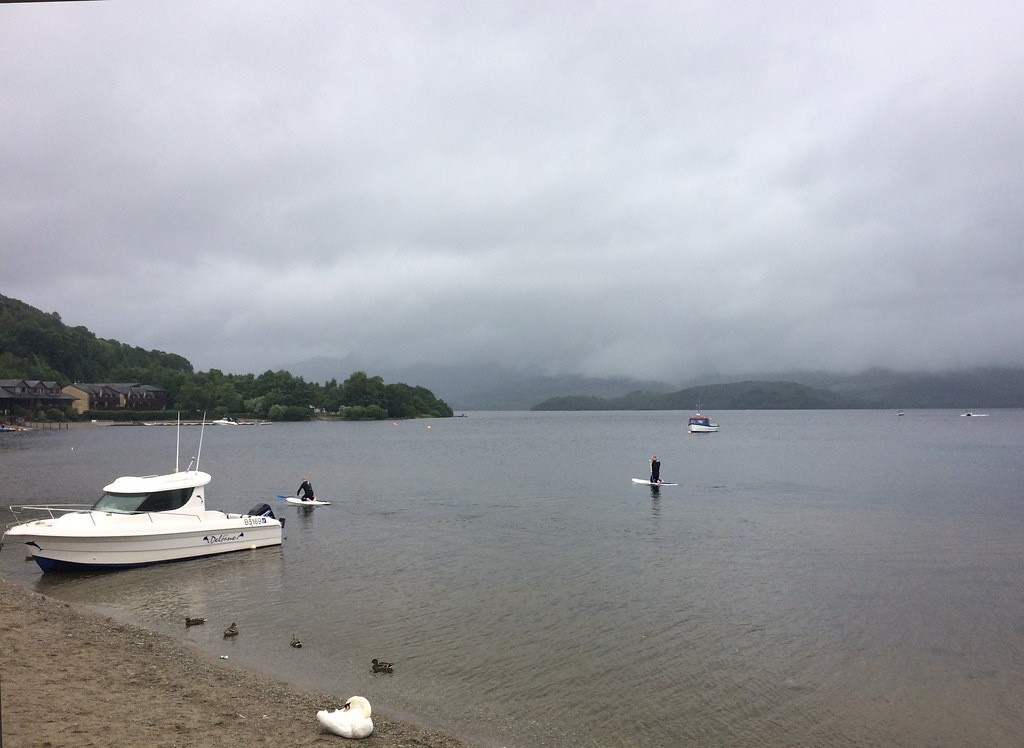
[688,394,720,433]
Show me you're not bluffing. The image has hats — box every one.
[651,455,656,460]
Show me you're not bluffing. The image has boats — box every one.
[144,422,213,426]
[260,420,272,425]
[3,410,287,574]
[0,425,16,431]
[960,410,990,417]
[238,421,254,425]
[213,415,237,425]
[896,409,905,416]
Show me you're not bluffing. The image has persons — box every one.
[296,476,316,501]
[649,455,661,482]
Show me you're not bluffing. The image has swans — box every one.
[317,695,373,740]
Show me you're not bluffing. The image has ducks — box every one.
[224,622,239,636]
[289,633,303,649]
[185,617,208,627]
[370,658,395,671]
